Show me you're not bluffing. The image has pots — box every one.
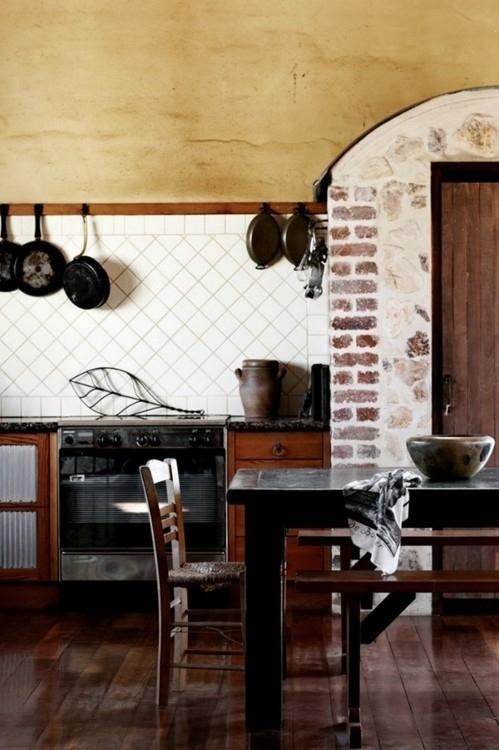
[242,204,316,267]
[0,200,113,309]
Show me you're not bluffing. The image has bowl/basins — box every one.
[408,434,495,482]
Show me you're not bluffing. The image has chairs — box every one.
[138,455,246,708]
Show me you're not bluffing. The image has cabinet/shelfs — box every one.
[226,423,332,613]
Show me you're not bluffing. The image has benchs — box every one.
[294,528,498,671]
[288,569,498,749]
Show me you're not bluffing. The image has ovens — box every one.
[52,413,229,598]
